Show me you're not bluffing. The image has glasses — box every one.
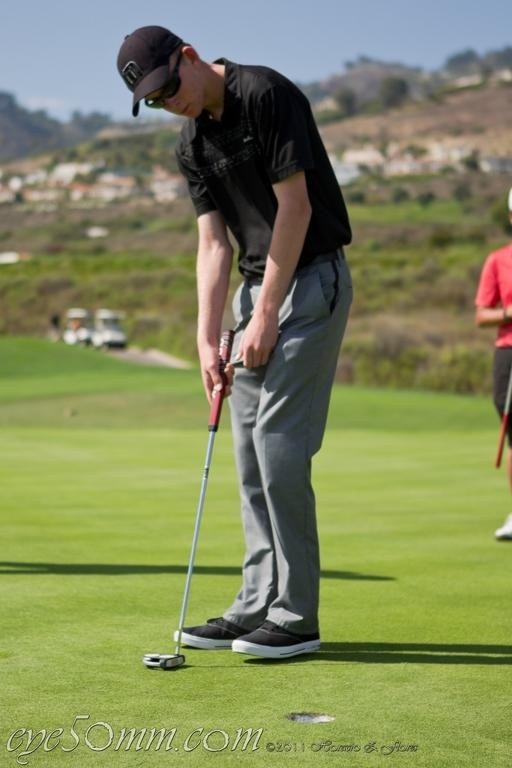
[146,48,183,109]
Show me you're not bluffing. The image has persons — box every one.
[116,24,354,660]
[473,184,512,540]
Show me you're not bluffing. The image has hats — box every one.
[117,26,183,116]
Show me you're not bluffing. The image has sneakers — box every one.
[231,621,323,659]
[496,515,512,539]
[176,618,250,650]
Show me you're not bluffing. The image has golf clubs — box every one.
[144,330,234,669]
